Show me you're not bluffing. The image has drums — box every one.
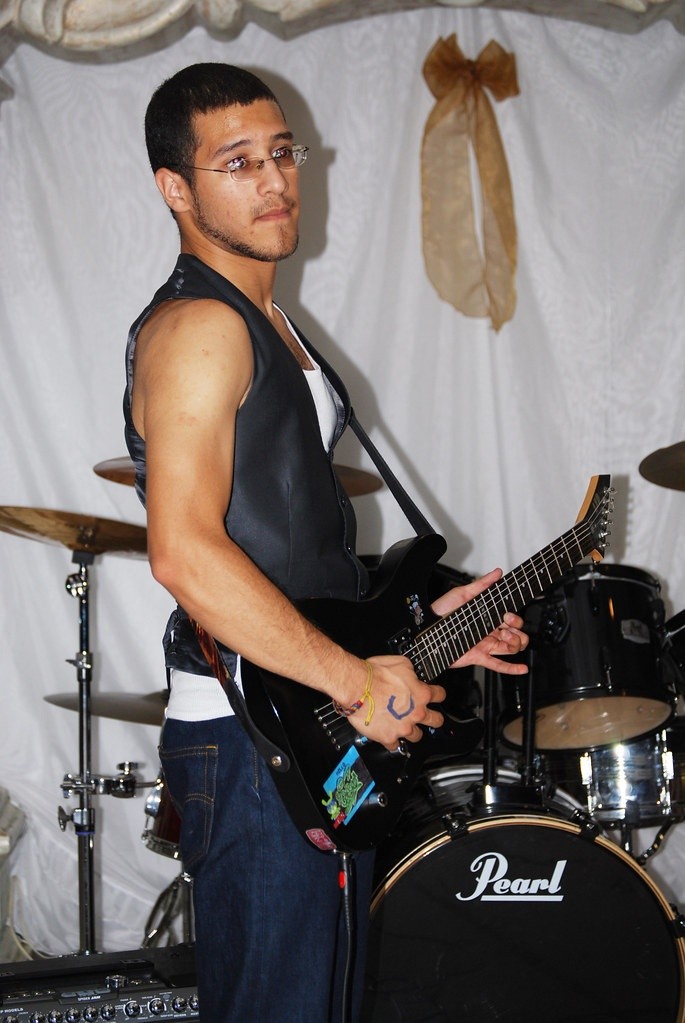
[141,766,185,860]
[499,564,678,756]
[353,762,685,1023]
[548,727,674,826]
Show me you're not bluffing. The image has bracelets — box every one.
[333,658,374,726]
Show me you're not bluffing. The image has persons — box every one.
[114,62,528,1022]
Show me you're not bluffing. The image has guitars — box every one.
[240,470,619,858]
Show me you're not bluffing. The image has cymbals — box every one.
[0,503,151,562]
[93,455,385,498]
[637,438,685,492]
[44,690,166,728]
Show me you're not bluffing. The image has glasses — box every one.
[165,144,310,181]
